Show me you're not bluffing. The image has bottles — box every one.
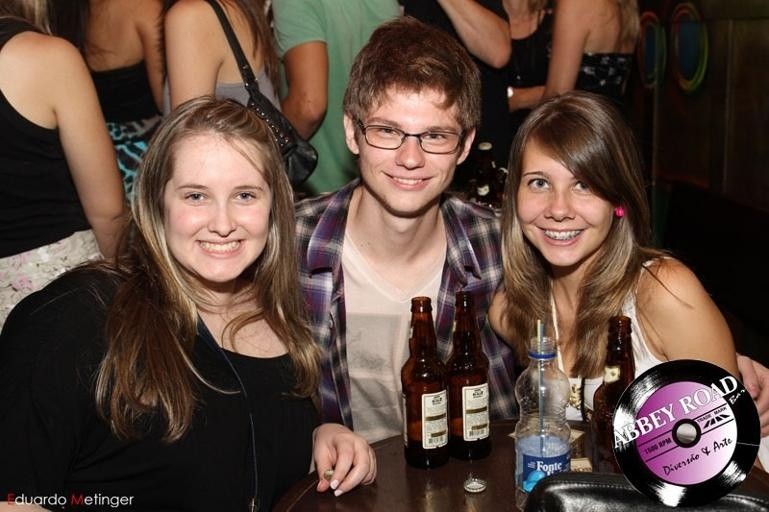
[590,314,637,473]
[515,337,572,510]
[447,293,493,457]
[401,297,450,468]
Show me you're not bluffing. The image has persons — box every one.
[293,16,768,452]
[2,92,379,511]
[487,91,768,478]
[0,0,666,327]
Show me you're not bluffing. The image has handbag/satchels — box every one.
[204,0,319,191]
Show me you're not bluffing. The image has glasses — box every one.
[350,111,468,155]
